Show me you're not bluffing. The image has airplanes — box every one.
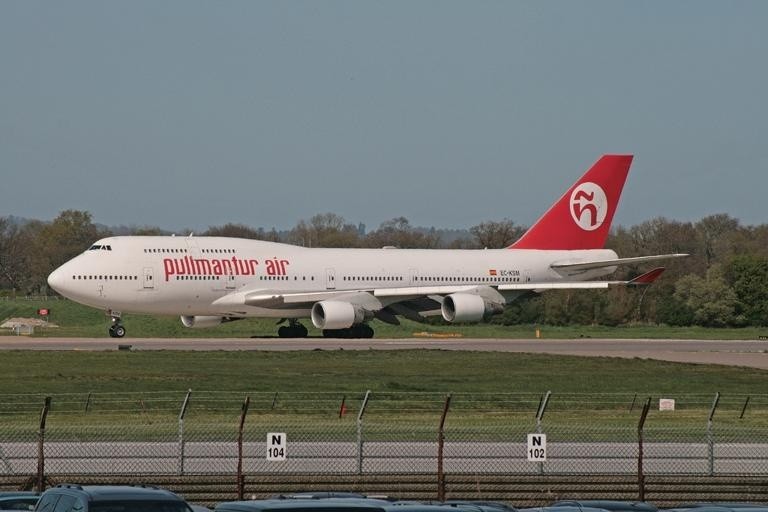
[47,153,692,338]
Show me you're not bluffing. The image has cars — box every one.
[1,484,768,512]
